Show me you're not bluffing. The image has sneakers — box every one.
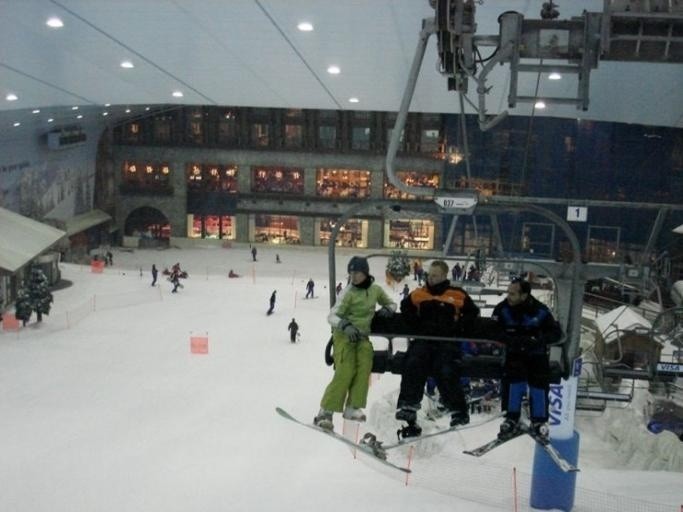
[395,405,418,421]
[527,419,552,446]
[498,414,520,436]
[342,405,367,422]
[449,410,470,427]
[313,408,334,429]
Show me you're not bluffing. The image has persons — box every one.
[316,256,398,429]
[385,261,475,300]
[151,264,157,286]
[393,259,480,426]
[492,277,564,438]
[306,278,314,299]
[169,262,184,293]
[252,247,257,261]
[337,283,343,295]
[288,318,298,343]
[267,290,277,315]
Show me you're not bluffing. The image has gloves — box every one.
[340,319,360,343]
[376,305,394,320]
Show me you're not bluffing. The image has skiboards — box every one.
[276,407,411,473]
[384,410,507,449]
[463,415,580,472]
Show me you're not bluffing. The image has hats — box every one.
[348,256,369,276]
